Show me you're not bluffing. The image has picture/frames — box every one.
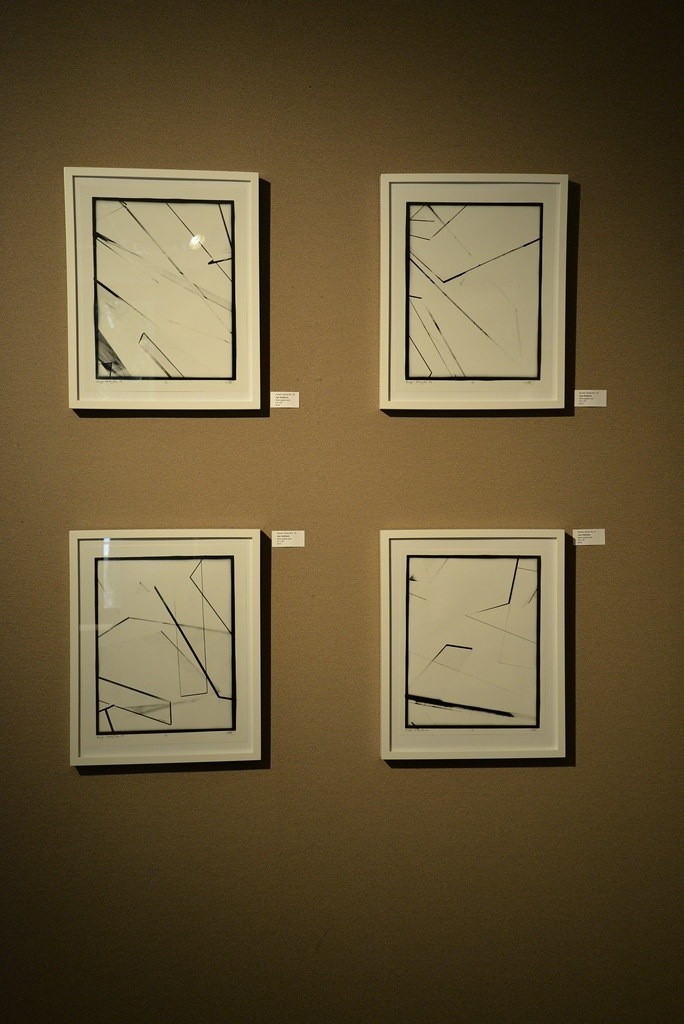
[62,165,262,410]
[69,529,265,767]
[379,529,565,759]
[378,172,570,410]
